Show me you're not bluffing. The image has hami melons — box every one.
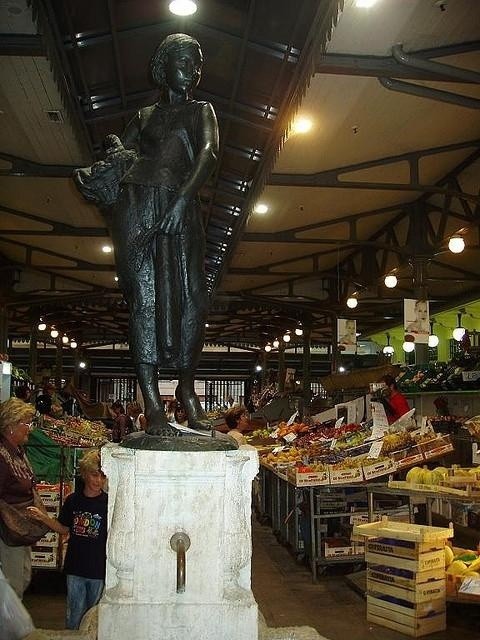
[445,546,479,581]
[407,467,447,485]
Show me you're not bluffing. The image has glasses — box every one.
[20,422,38,431]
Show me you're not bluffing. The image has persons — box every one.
[14,386,30,402]
[225,406,249,445]
[166,399,189,428]
[110,402,146,443]
[36,390,72,418]
[23,450,112,630]
[0,396,51,620]
[406,298,430,336]
[379,375,411,426]
[73,35,225,438]
[339,320,356,346]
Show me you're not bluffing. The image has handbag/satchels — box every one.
[0,486,50,547]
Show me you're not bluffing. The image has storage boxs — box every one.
[30,482,73,568]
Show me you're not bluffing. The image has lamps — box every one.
[35,322,77,350]
[244,229,469,355]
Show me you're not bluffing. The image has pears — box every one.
[261,447,302,463]
